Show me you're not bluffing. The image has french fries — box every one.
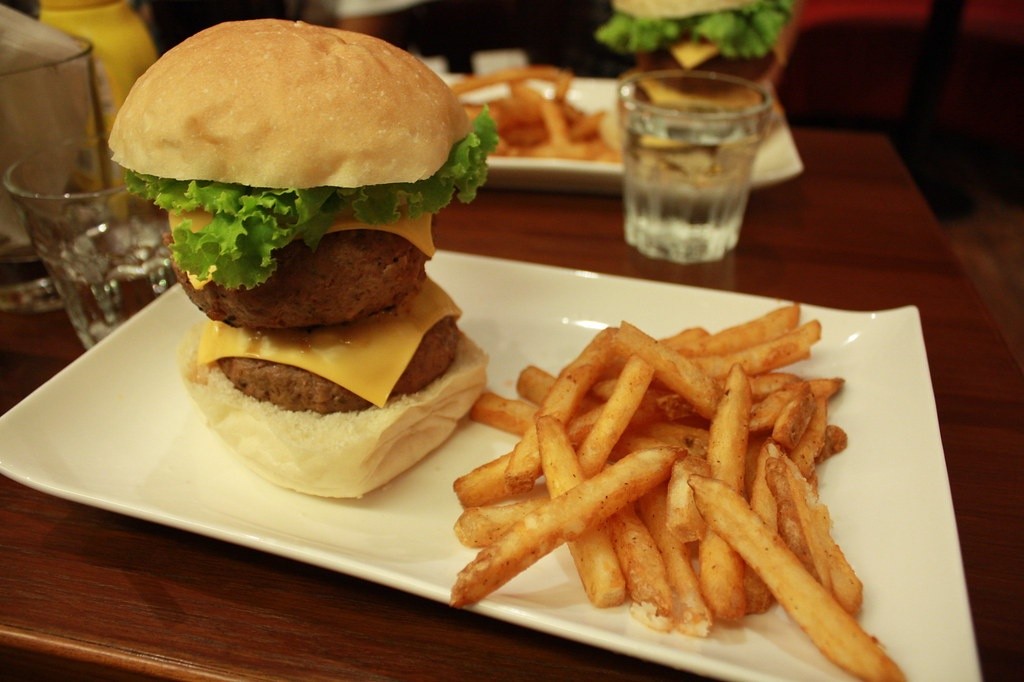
[439,303,904,681]
[446,65,620,163]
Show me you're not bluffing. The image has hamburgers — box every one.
[596,0,792,147]
[107,16,505,498]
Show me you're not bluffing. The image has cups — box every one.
[0,32,110,312]
[4,135,179,348]
[617,73,772,263]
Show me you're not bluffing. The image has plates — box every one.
[0,253,980,682]
[442,73,805,192]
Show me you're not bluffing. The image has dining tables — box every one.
[0,128,1024,682]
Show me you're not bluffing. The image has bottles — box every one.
[41,0,157,219]
